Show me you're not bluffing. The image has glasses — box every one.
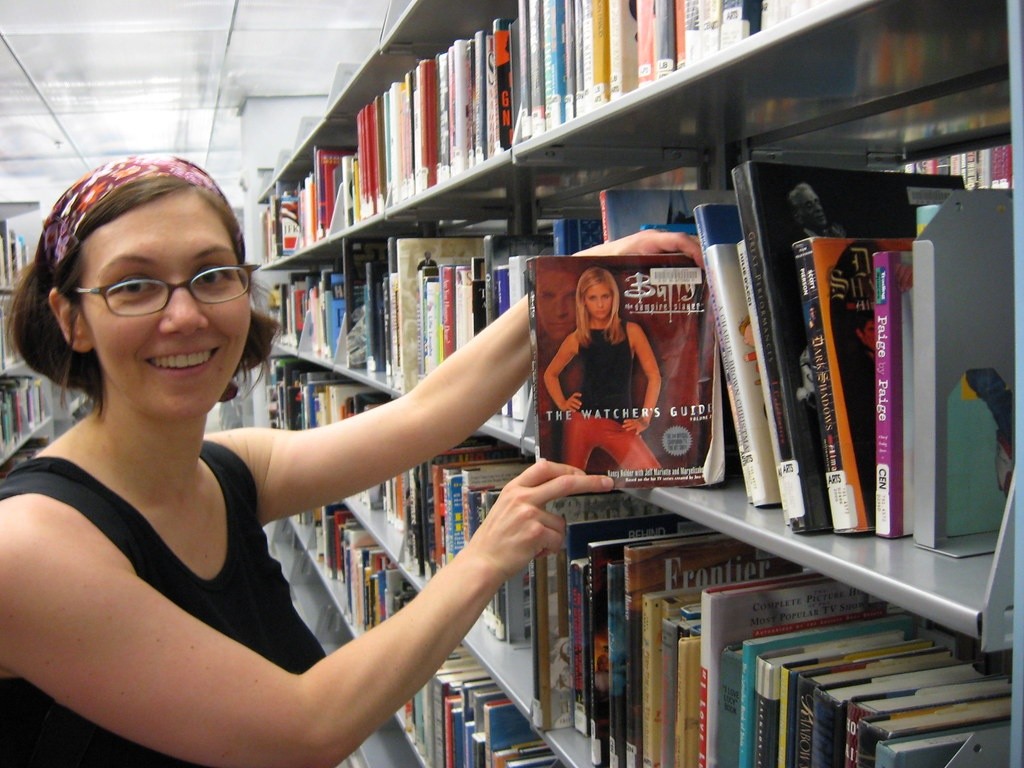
[75,265,250,316]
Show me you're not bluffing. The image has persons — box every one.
[536,258,580,340]
[1,154,705,767]
[544,267,662,475]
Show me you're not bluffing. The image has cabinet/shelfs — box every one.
[0,285,96,483]
[257,0,1023,767]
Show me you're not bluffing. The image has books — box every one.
[262,353,1013,768]
[1,218,50,480]
[266,143,1011,539]
[257,0,820,258]
